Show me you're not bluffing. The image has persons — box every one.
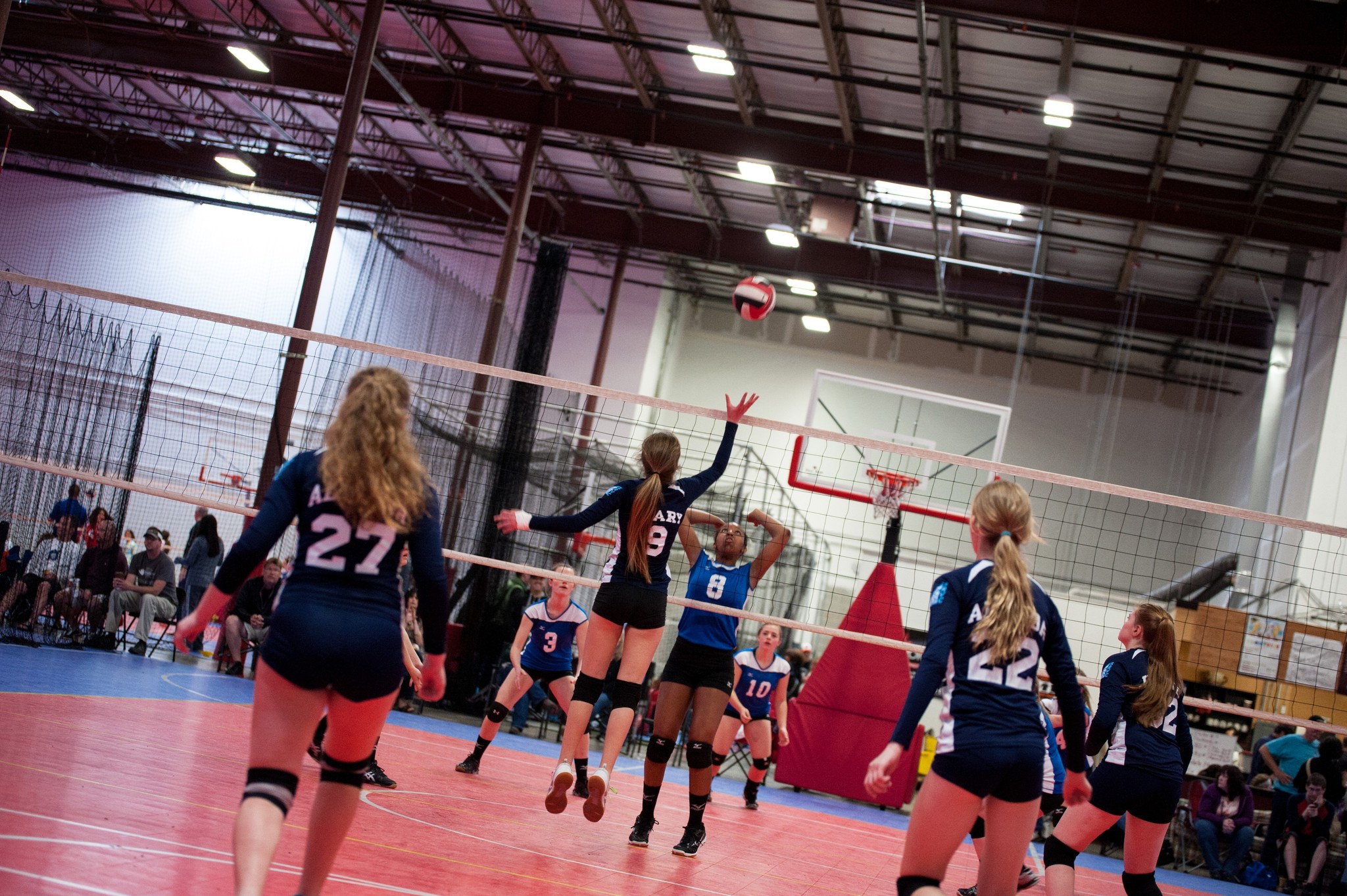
[864,478,1093,896]
[225,558,282,676]
[178,514,224,656]
[1043,604,1193,896]
[0,483,815,781]
[493,390,759,823]
[1195,766,1254,880]
[86,527,178,655]
[1036,668,1347,896]
[708,623,791,809]
[456,562,595,799]
[955,697,1066,896]
[627,507,791,857]
[1280,772,1336,896]
[175,365,450,896]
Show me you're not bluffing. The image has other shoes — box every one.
[1281,878,1317,896]
[72,630,85,644]
[225,661,243,675]
[543,698,557,714]
[17,621,34,632]
[52,620,63,629]
[957,865,1042,896]
[583,763,610,823]
[84,631,116,653]
[743,788,759,810]
[545,757,573,814]
[508,727,520,734]
[128,640,146,656]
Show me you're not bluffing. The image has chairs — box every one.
[482,643,766,785]
[114,588,260,673]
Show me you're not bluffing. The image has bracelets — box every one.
[181,564,189,571]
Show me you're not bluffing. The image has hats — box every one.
[143,529,163,541]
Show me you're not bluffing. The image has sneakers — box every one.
[456,751,480,774]
[628,810,655,846]
[306,739,398,789]
[672,822,707,857]
[572,775,589,797]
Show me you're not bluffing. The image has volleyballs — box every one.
[734,277,778,321]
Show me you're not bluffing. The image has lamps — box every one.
[766,223,799,249]
[213,152,258,177]
[1044,92,1074,118]
[227,42,271,74]
